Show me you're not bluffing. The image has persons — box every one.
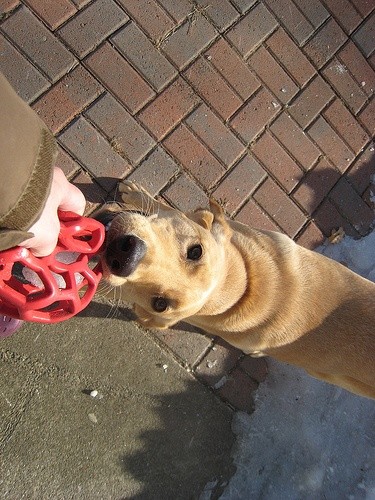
[0,71,86,256]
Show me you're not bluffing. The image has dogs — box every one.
[102,180,374,402]
